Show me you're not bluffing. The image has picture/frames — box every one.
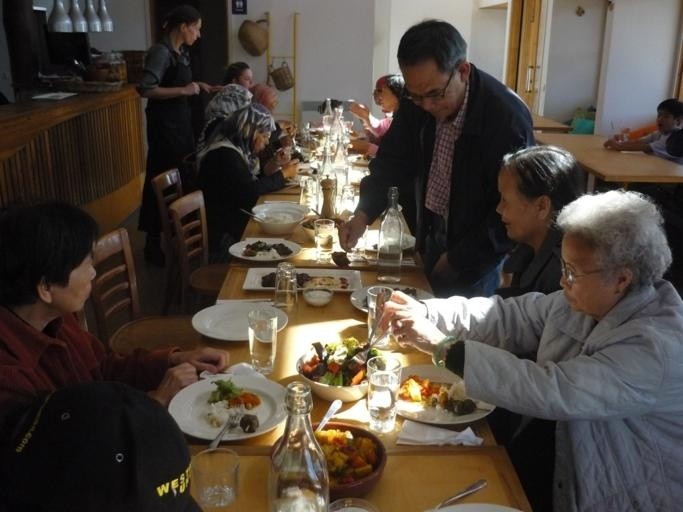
[232,0,247,15]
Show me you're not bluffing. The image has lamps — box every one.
[47,1,114,34]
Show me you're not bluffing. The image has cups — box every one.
[275,261,299,312]
[314,218,336,265]
[366,355,403,434]
[248,309,277,375]
[340,184,355,212]
[299,175,316,214]
[327,497,378,512]
[367,286,393,347]
[194,448,241,512]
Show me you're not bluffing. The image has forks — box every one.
[344,328,392,372]
[208,404,241,452]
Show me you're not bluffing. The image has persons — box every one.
[350,75,404,137]
[374,75,388,106]
[603,98,683,159]
[248,83,296,161]
[495,146,582,293]
[339,19,534,285]
[136,6,214,261]
[384,189,683,511]
[182,103,300,242]
[224,61,253,92]
[0,200,229,511]
[206,84,254,121]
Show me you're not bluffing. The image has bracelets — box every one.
[432,336,458,368]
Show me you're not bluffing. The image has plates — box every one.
[350,166,372,185]
[350,283,436,314]
[433,502,524,512]
[167,375,288,441]
[228,238,302,262]
[192,301,289,342]
[349,154,371,167]
[395,364,498,425]
[358,229,416,253]
[251,204,309,215]
[242,267,361,293]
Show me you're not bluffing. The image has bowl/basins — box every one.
[253,205,304,234]
[271,422,387,504]
[295,352,369,403]
[299,217,316,241]
[302,286,334,307]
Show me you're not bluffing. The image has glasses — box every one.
[401,70,455,101]
[559,258,627,285]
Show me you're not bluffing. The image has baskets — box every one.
[270,62,293,91]
[238,19,268,56]
[43,79,123,92]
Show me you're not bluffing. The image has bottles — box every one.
[329,108,345,144]
[268,381,330,512]
[323,132,331,146]
[322,96,333,132]
[376,186,404,283]
[331,133,348,196]
[315,145,338,212]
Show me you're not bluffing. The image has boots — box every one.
[144,234,165,268]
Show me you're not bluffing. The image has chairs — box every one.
[80,226,200,359]
[72,309,88,331]
[172,191,228,314]
[149,168,185,258]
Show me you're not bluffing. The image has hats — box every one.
[1,382,191,512]
